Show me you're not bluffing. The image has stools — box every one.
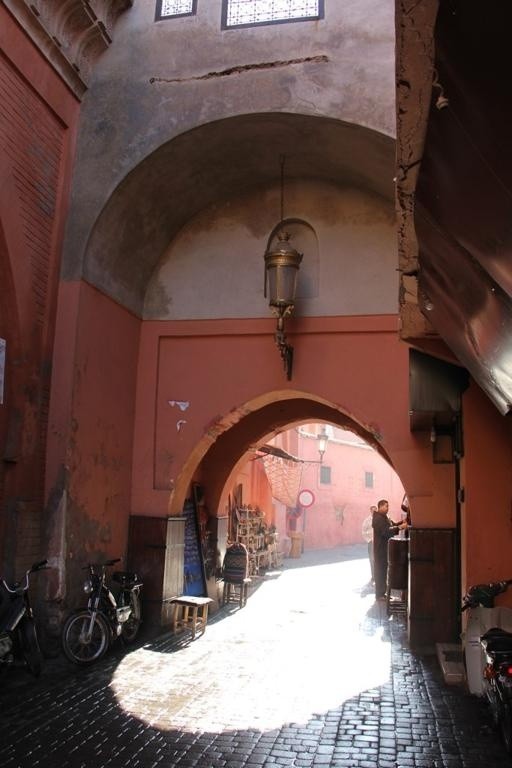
[169,595,213,641]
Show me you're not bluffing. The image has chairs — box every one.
[221,542,252,609]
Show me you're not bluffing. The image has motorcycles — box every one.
[61,558,144,666]
[460,578,512,753]
[0,559,51,679]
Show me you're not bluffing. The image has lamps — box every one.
[263,229,305,381]
[303,428,328,464]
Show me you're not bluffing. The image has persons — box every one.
[372,501,407,602]
[362,505,376,586]
